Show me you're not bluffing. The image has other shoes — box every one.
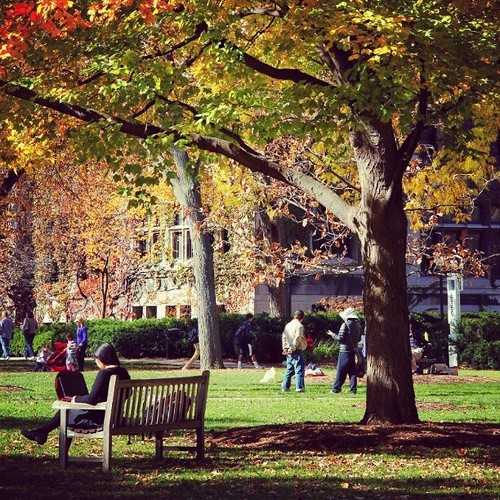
[29,356,34,361]
[296,388,304,392]
[282,389,290,392]
[22,430,46,445]
[6,356,9,360]
[331,390,337,394]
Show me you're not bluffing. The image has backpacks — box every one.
[235,321,250,338]
[188,328,198,341]
[139,391,191,422]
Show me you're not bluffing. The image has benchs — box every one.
[53,370,210,473]
[416,358,448,374]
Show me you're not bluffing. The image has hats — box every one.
[339,307,360,321]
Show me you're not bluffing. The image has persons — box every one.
[409,317,426,375]
[22,342,132,469]
[20,312,38,361]
[237,313,264,369]
[65,333,79,371]
[282,309,307,393]
[33,343,51,372]
[327,308,364,395]
[0,311,14,360]
[182,324,200,370]
[76,318,88,372]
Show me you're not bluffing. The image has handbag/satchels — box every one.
[355,351,362,365]
[23,317,30,332]
